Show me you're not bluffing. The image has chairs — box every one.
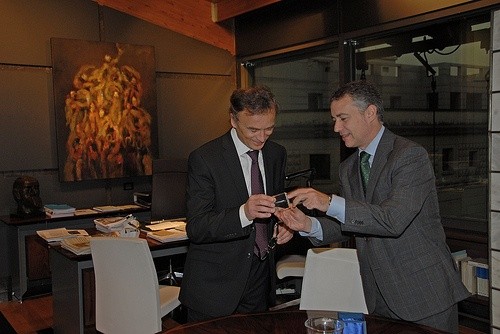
[269,240,369,315]
[89,172,190,334]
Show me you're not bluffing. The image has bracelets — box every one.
[329,195,332,207]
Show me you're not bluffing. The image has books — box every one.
[37,228,107,255]
[44,204,76,218]
[94,217,127,233]
[147,229,189,243]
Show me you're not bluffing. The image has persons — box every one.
[273,79,472,334]
[177,85,293,322]
[13,176,43,215]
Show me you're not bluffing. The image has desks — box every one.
[0,201,153,302]
[36,217,189,334]
[160,311,451,334]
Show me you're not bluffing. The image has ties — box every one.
[358,151,371,199]
[246,150,268,260]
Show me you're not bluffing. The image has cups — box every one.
[305,316,344,334]
